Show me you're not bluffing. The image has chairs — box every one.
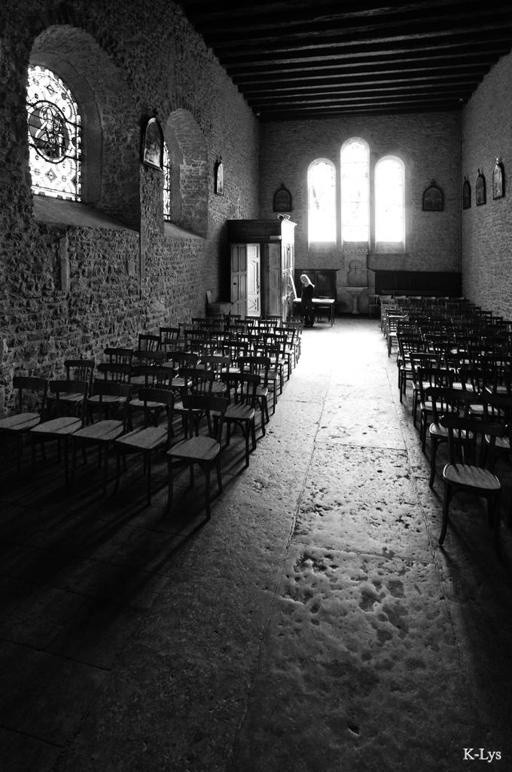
[379,296,512,545]
[0,313,304,521]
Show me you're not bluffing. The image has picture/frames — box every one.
[273,182,292,212]
[140,109,163,171]
[214,155,224,195]
[422,157,505,212]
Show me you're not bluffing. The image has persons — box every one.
[299,274,315,328]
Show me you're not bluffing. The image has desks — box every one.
[292,298,335,327]
